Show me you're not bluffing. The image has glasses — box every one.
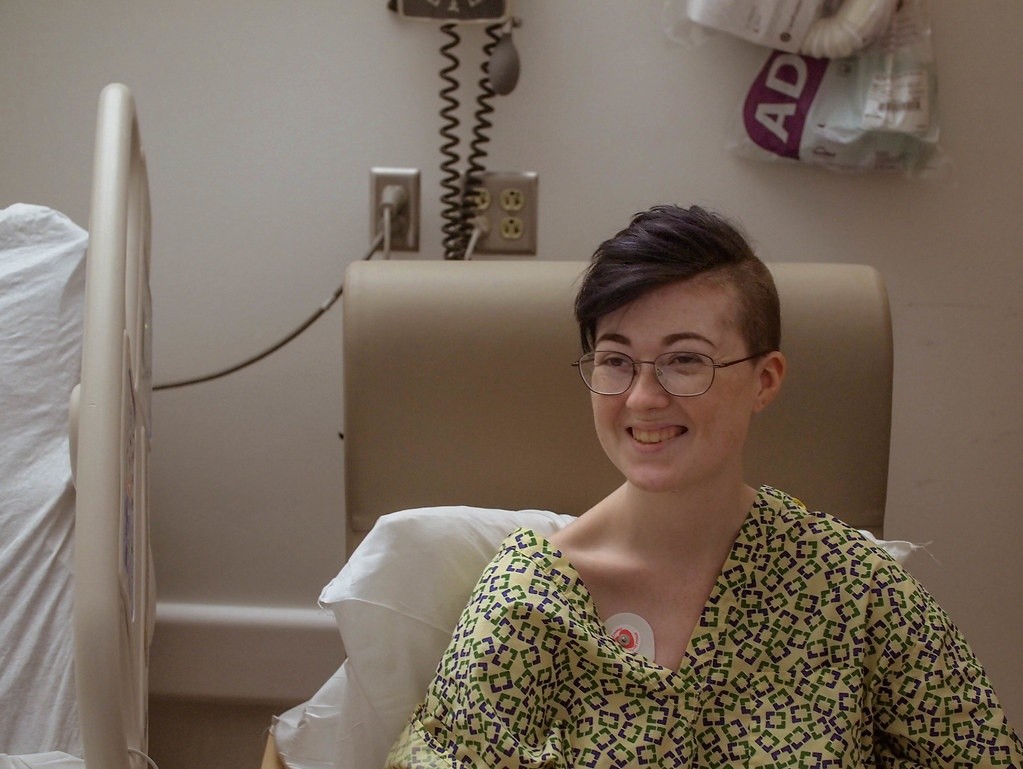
[572,350,773,398]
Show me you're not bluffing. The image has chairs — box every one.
[339,257,901,755]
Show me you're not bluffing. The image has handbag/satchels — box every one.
[738,0,943,175]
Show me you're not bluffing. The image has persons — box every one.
[381,206,1023,769]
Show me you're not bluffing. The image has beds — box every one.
[3,82,164,769]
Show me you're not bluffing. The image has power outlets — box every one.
[463,170,539,256]
[369,164,419,253]
